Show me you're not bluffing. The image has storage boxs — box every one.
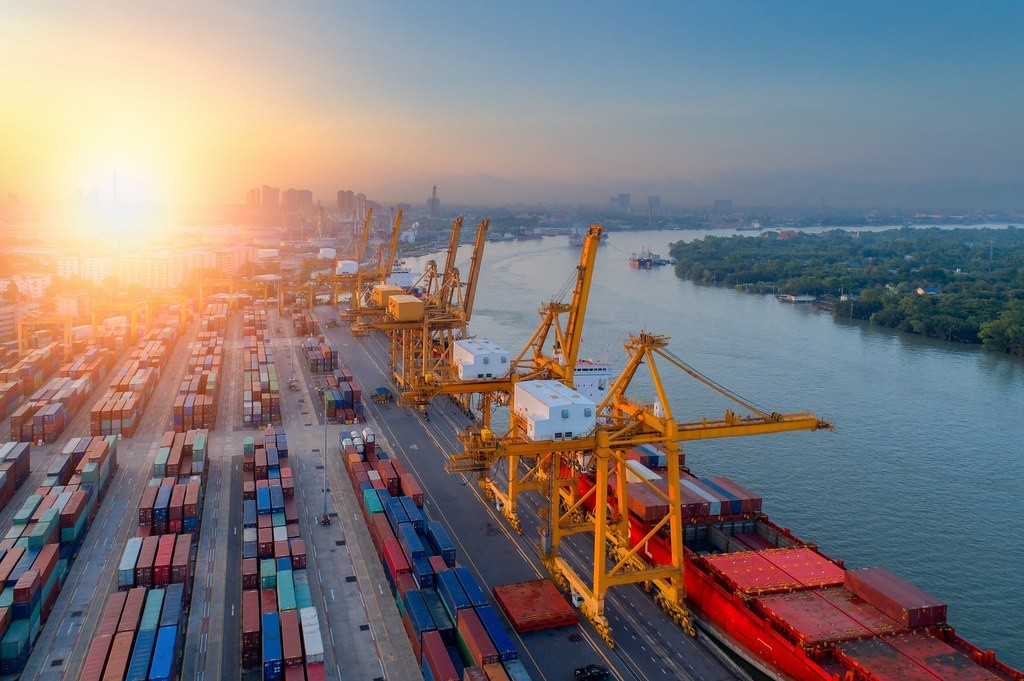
[0,287,1020,680]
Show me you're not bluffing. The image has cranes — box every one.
[312,202,838,648]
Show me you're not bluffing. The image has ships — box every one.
[505,356,1024,681]
